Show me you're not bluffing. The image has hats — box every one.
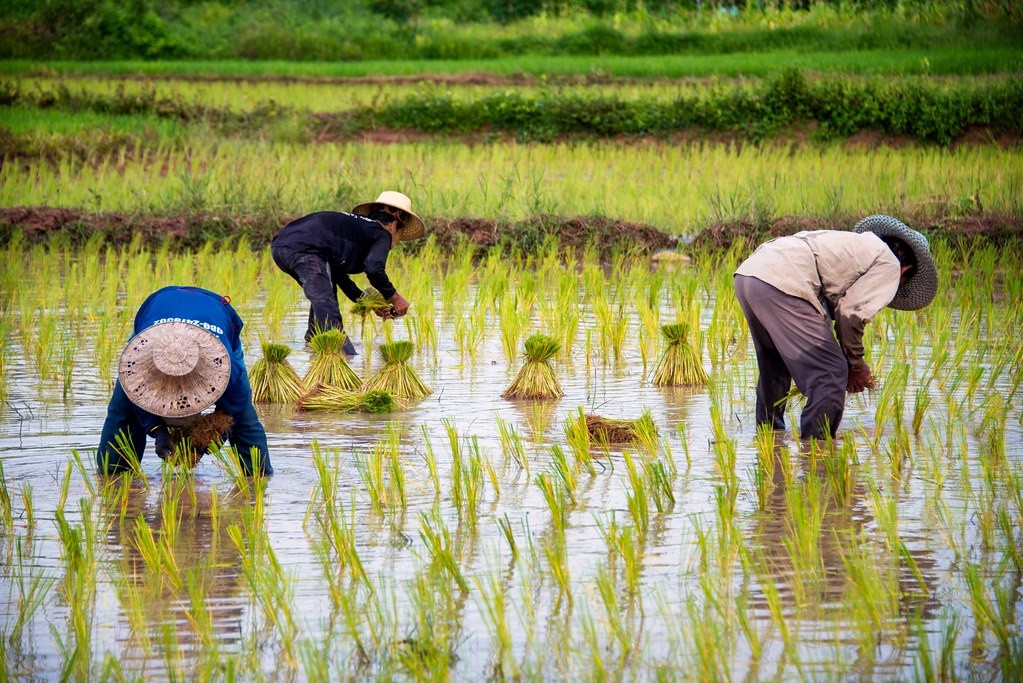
[119,323,231,417]
[352,191,425,240]
[853,215,938,311]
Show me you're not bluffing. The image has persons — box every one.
[270,191,426,354]
[96,284,274,479]
[731,214,939,441]
